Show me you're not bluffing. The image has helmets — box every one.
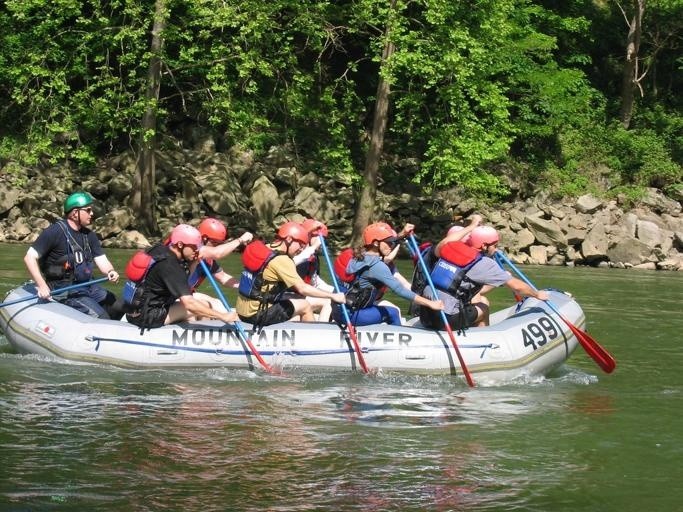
[447,226,499,250]
[278,222,309,244]
[362,222,400,246]
[199,217,227,243]
[302,219,328,238]
[64,190,97,214]
[170,224,201,244]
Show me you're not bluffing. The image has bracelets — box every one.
[107,269,115,274]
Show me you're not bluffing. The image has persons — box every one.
[429,215,512,307]
[420,226,549,331]
[235,222,346,327]
[374,230,411,323]
[291,219,336,300]
[22,191,124,320]
[183,217,253,313]
[122,224,241,335]
[330,222,443,327]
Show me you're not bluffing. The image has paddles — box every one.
[197,255,276,376]
[495,248,616,374]
[317,228,370,374]
[409,231,475,386]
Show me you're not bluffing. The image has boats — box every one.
[0,274,589,379]
